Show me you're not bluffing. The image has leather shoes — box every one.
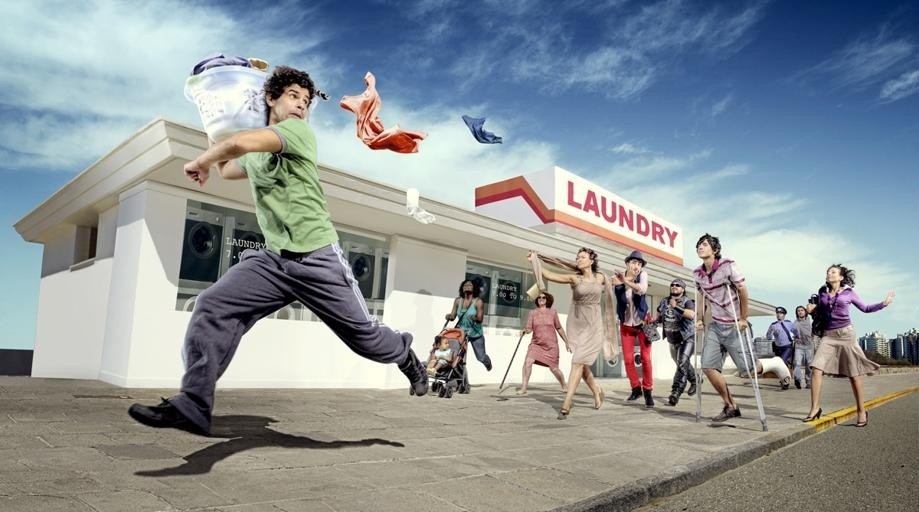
[126,396,208,436]
[668,392,679,406]
[688,378,703,396]
[399,347,429,395]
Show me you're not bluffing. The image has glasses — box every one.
[538,296,546,299]
[671,283,679,287]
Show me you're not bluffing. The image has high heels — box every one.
[855,410,869,427]
[803,407,822,422]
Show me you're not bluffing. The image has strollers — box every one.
[409,317,474,398]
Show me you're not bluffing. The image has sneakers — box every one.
[516,390,528,394]
[644,391,654,407]
[712,406,741,422]
[486,357,492,371]
[562,384,568,392]
[627,388,643,401]
[426,367,436,375]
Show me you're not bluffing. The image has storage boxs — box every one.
[183,65,318,144]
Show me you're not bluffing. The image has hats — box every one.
[776,307,787,314]
[624,251,647,267]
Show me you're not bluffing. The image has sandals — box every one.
[558,401,573,414]
[594,388,604,408]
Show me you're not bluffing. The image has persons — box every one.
[610,250,654,408]
[445,279,491,391]
[516,291,573,395]
[527,247,635,420]
[791,306,814,390]
[692,234,792,423]
[802,264,895,428]
[426,336,453,377]
[653,279,703,405]
[767,306,802,368]
[128,67,429,436]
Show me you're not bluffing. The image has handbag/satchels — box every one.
[643,314,660,342]
